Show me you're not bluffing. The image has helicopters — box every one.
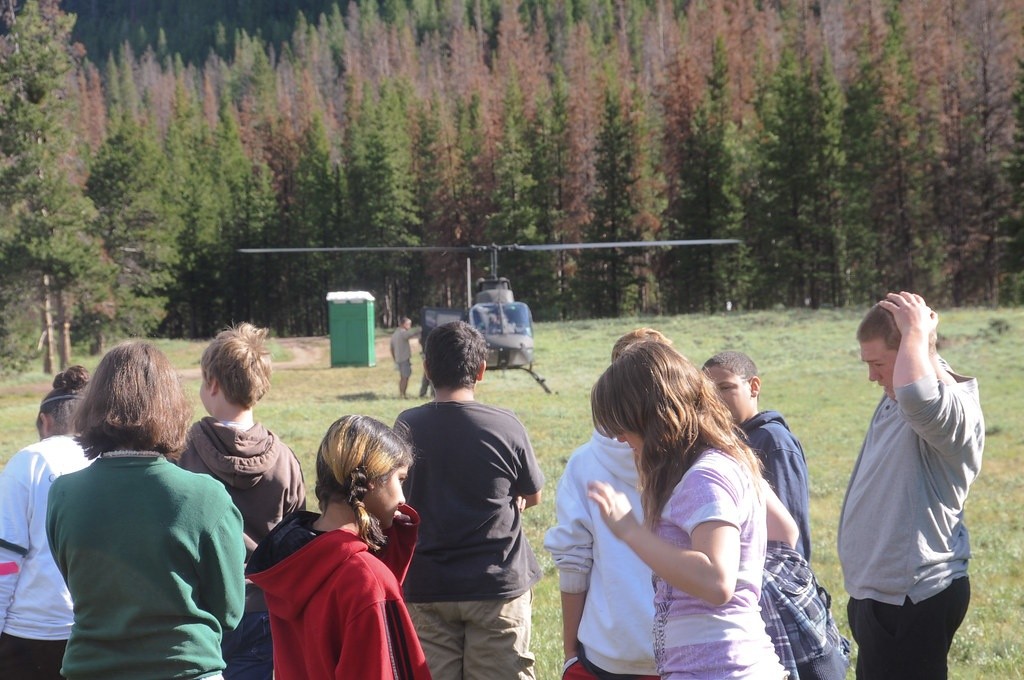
[234,239,742,396]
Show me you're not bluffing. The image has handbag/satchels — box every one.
[754,543,833,666]
[800,619,854,680]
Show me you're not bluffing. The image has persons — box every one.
[392,320,545,680]
[541,330,676,680]
[589,339,802,680]
[836,289,985,680]
[170,320,307,680]
[419,319,437,398]
[46,340,245,679]
[487,313,501,334]
[698,350,817,680]
[0,364,102,680]
[389,316,423,400]
[243,415,433,680]
